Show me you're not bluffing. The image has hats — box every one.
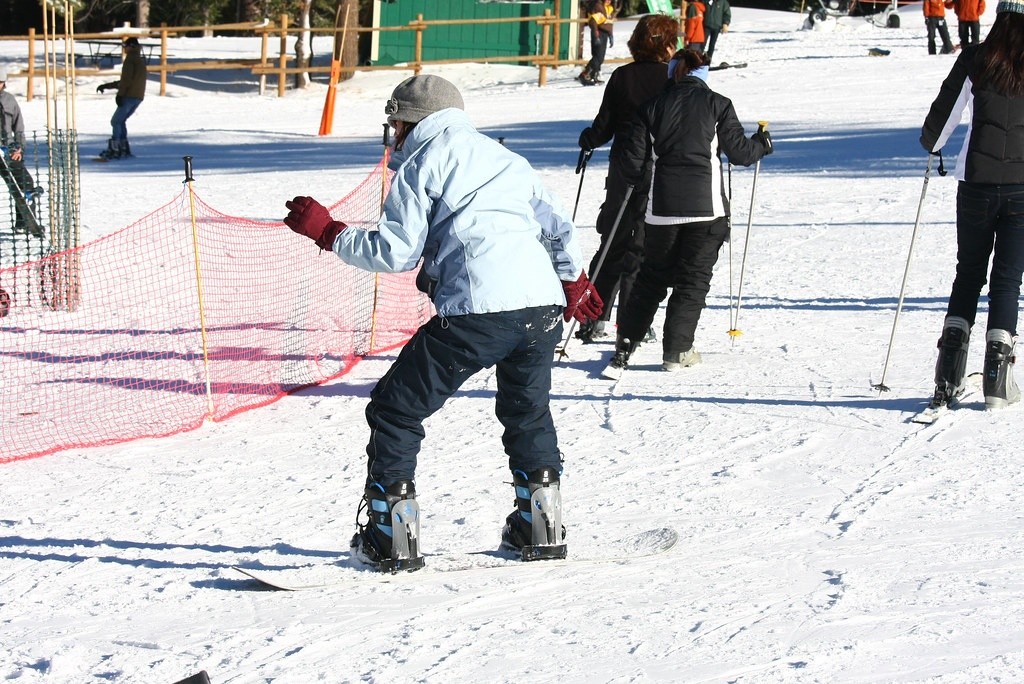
[385,76,464,127]
[996,0,1024,15]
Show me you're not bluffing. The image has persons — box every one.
[96,37,147,158]
[919,0,1024,402]
[0,65,46,234]
[578,9,682,343]
[575,0,613,85]
[922,0,987,55]
[283,74,604,574]
[597,49,774,363]
[685,0,731,59]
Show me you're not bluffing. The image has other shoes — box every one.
[641,330,657,342]
[608,348,632,369]
[579,317,605,337]
[121,149,132,158]
[15,223,46,234]
[940,47,955,54]
[662,344,696,372]
[99,146,122,159]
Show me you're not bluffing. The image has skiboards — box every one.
[601,360,624,380]
[909,371,983,424]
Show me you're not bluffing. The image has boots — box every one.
[983,328,1021,409]
[932,315,971,407]
[361,481,418,569]
[501,468,567,559]
[590,71,605,85]
[579,65,595,85]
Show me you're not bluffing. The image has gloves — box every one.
[559,268,604,325]
[751,131,773,157]
[593,38,601,45]
[578,127,592,151]
[283,195,347,251]
[609,36,613,48]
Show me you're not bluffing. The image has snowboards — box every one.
[0,148,44,239]
[92,154,136,162]
[229,521,681,592]
